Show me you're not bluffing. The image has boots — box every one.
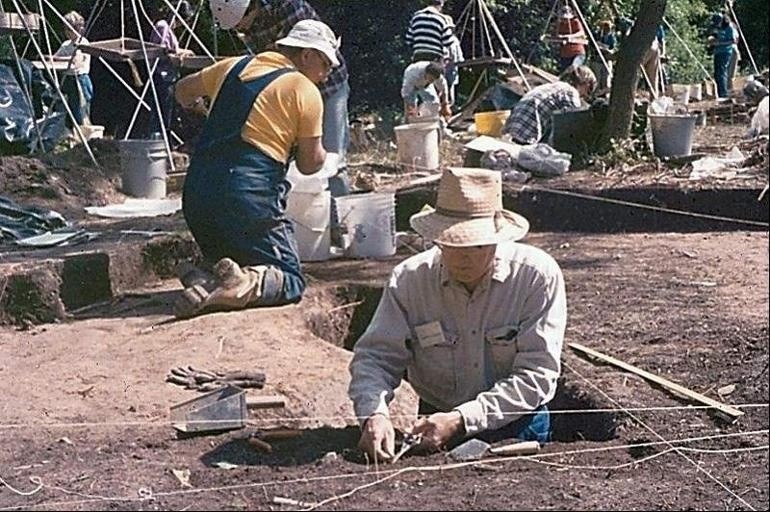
[173,257,286,319]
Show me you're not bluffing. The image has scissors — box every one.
[391,433,421,465]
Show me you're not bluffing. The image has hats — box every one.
[408,165,529,249]
[274,19,343,68]
[554,5,578,20]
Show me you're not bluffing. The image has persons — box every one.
[706,14,740,90]
[167,17,343,322]
[398,2,669,137]
[703,16,736,98]
[47,9,96,130]
[345,167,570,463]
[206,0,353,170]
[144,1,196,139]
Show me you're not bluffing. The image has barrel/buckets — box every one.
[392,121,442,169]
[118,138,170,198]
[474,110,511,137]
[286,191,332,262]
[648,103,698,160]
[335,191,398,257]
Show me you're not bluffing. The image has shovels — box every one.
[447,438,541,463]
